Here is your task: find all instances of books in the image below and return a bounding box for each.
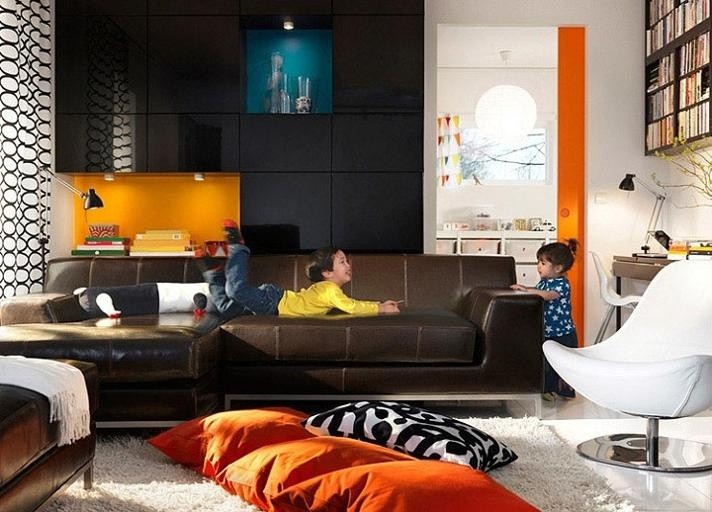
[646,0,710,151]
[667,239,712,261]
[71,230,195,256]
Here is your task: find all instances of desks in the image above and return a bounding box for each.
[611,254,677,333]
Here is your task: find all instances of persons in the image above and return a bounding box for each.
[191,218,400,320]
[510,238,580,402]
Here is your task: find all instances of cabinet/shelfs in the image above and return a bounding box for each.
[436,231,557,289]
[56,2,423,254]
[643,1,711,157]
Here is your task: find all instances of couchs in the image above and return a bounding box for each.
[0,254,545,428]
[0,354,97,511]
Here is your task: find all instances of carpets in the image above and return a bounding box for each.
[36,414,640,512]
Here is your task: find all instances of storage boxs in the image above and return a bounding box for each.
[504,239,544,262]
[461,239,500,254]
[89,223,120,238]
[437,238,457,254]
[515,265,542,287]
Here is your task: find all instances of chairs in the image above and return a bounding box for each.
[541,258,710,474]
[587,250,642,344]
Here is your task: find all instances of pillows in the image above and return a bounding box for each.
[214,435,420,512]
[297,397,519,472]
[146,406,317,479]
[267,458,540,511]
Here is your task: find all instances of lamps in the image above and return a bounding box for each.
[475,48,538,140]
[618,172,670,259]
[40,165,104,287]
